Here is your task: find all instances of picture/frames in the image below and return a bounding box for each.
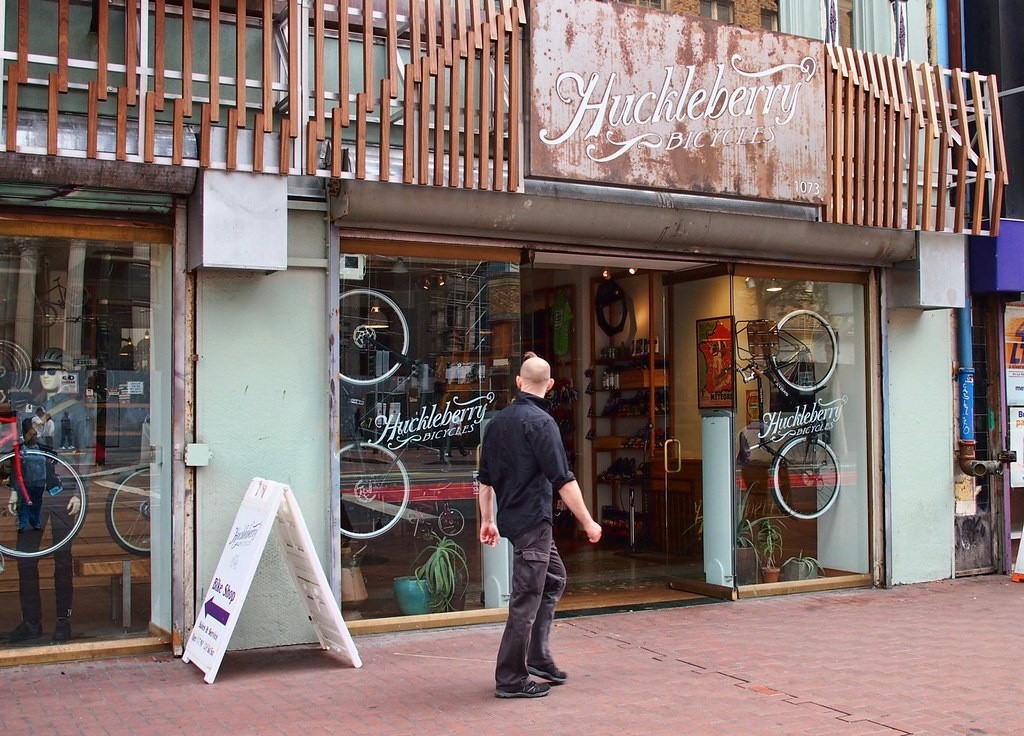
[695,315,737,408]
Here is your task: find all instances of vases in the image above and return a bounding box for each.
[342,566,369,620]
[446,574,470,610]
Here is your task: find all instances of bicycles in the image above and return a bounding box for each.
[406,481,465,540]
[23,276,90,328]
[0,393,88,561]
[105,465,151,557]
[715,309,841,522]
[340,288,465,541]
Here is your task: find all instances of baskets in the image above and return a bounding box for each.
[736,319,779,362]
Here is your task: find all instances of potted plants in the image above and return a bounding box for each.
[780,548,826,580]
[755,514,784,584]
[736,475,759,584]
[391,529,472,617]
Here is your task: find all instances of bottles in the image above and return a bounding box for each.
[603,372,619,390]
[601,336,659,358]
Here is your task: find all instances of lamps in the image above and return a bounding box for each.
[745,276,756,289]
[371,298,381,313]
[628,266,639,275]
[438,275,446,286]
[365,308,389,330]
[765,279,783,292]
[423,277,432,290]
[601,269,612,280]
[390,257,409,275]
[804,281,814,292]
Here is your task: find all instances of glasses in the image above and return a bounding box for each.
[37,368,68,376]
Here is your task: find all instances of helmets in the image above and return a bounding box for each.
[35,347,73,369]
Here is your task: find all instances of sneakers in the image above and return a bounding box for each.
[494,680,550,698]
[528,661,568,682]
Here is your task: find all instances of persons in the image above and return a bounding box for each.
[13,406,75,532]
[476,357,602,698]
[441,378,473,457]
[0,346,94,643]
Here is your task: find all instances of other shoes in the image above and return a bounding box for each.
[462,452,469,457]
[9,620,43,640]
[60,446,75,450]
[53,616,71,640]
[16,524,41,530]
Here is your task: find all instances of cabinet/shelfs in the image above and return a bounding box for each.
[647,453,817,564]
[590,353,670,548]
